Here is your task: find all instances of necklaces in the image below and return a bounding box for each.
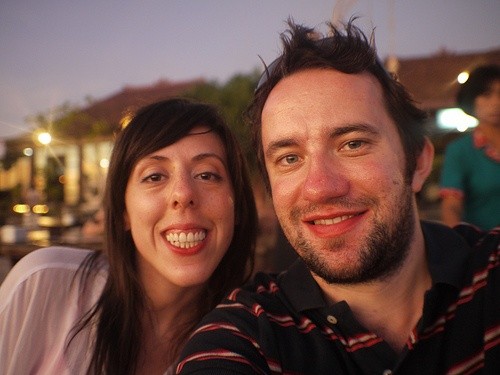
[139,289,203,326]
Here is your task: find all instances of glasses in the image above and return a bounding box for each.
[253,34,390,95]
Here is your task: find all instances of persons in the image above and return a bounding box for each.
[436,67,500,231]
[169,16,499,374]
[1,99,274,374]
